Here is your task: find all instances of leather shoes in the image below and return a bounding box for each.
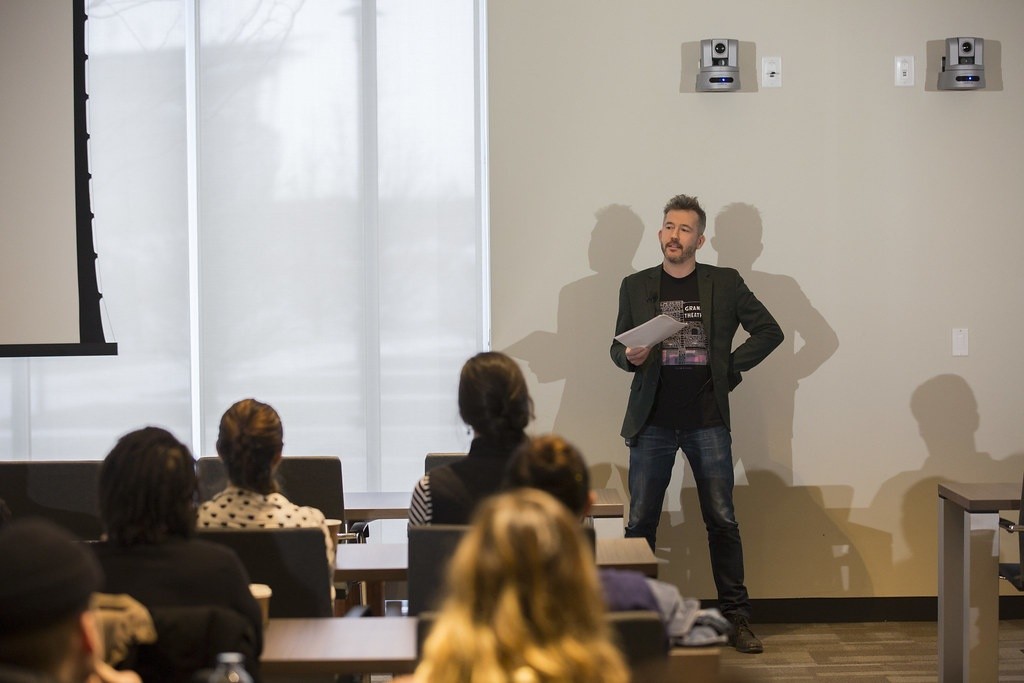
[724,609,767,655]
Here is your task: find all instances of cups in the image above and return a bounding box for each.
[248,584,272,631]
[327,519,342,555]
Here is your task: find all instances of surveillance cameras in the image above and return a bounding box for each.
[937,37,986,90]
[696,38,741,92]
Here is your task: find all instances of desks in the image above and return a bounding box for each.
[333,537,660,584]
[937,481,1024,683]
[343,487,626,525]
[258,617,722,683]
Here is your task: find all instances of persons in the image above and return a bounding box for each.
[406,352,530,534]
[85,427,264,683]
[500,437,660,612]
[196,398,336,610]
[0,521,141,683]
[609,193,784,655]
[413,488,628,682]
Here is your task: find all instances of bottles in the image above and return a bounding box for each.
[209,652,254,683]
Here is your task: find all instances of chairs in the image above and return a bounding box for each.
[0,459,105,540]
[118,605,262,683]
[195,526,334,618]
[196,457,346,533]
[415,608,670,683]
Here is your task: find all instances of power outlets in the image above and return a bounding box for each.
[893,55,914,87]
[762,55,782,88]
